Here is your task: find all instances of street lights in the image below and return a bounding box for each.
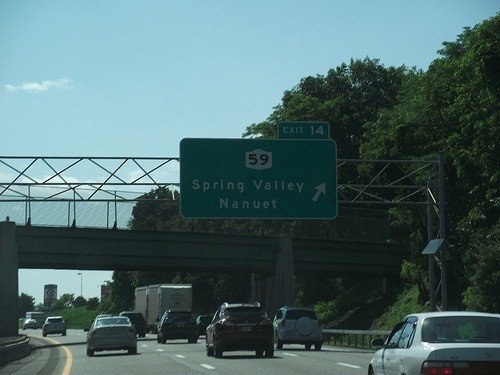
[77,273,83,297]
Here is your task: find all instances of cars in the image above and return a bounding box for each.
[196,316,212,338]
[119,312,146,337]
[155,309,199,344]
[84,316,137,356]
[206,302,274,358]
[368,312,500,375]
[43,316,67,337]
[23,318,37,330]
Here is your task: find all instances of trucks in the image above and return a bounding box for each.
[135,285,193,334]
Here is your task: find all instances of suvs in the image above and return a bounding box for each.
[273,305,323,351]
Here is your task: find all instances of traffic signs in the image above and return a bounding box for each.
[179,121,338,220]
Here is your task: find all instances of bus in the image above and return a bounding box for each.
[26,312,45,329]
